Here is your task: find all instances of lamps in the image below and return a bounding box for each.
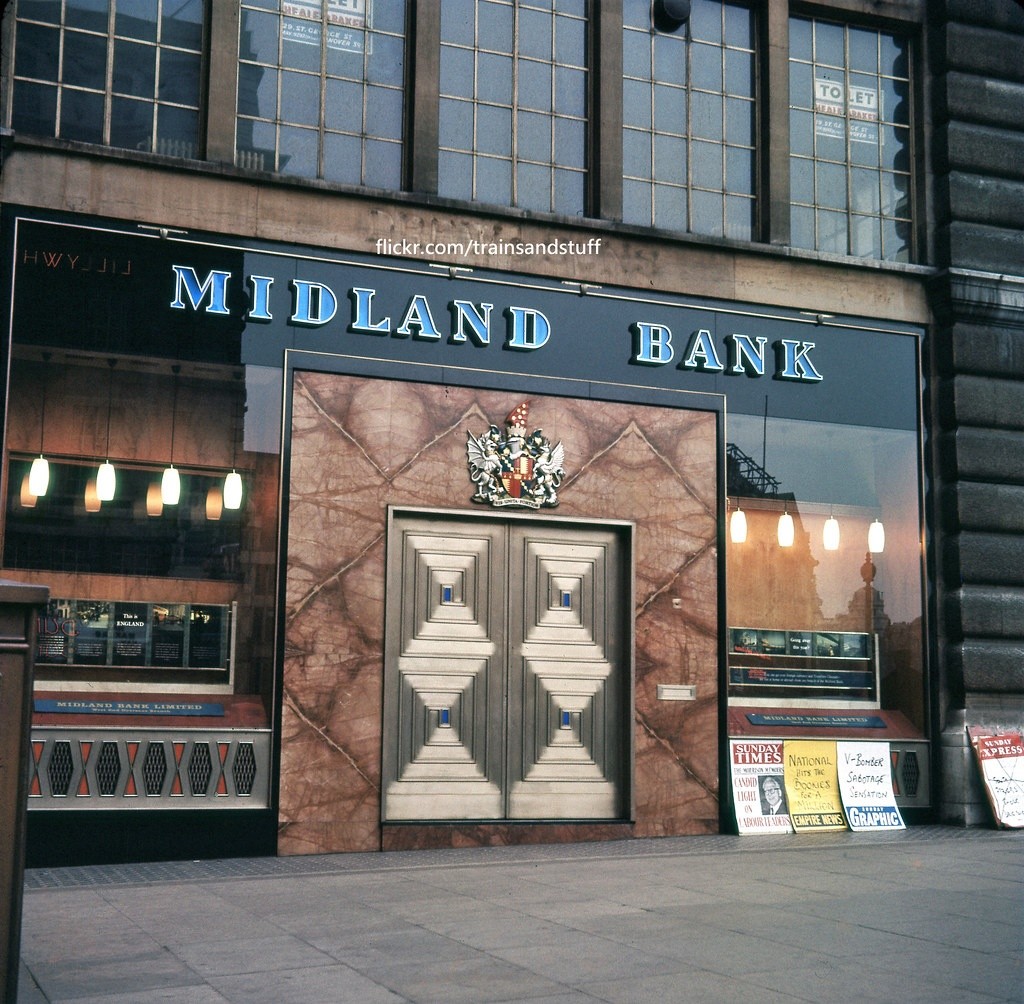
[730,491,887,554]
[16,352,247,521]
